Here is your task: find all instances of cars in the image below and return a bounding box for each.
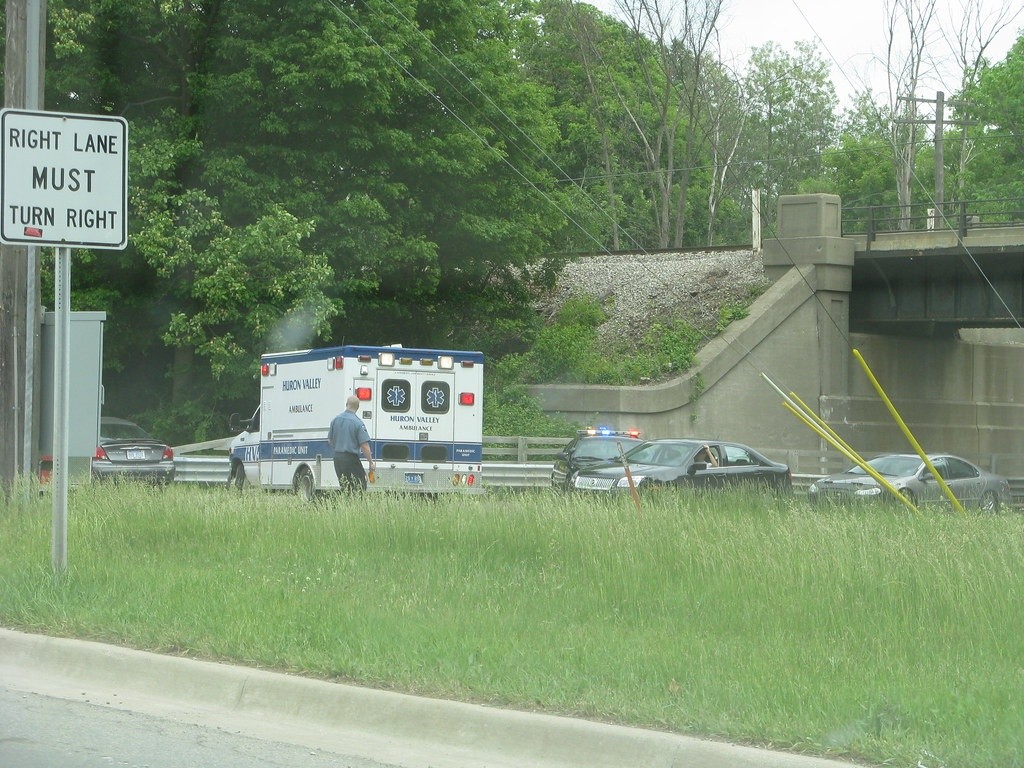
[93,416,175,486]
[570,438,793,506]
[551,426,645,494]
[808,455,1010,516]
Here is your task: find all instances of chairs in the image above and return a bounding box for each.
[737,458,751,466]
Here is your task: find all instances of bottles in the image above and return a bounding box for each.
[368,470,375,483]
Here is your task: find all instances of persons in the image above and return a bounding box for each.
[327,395,374,501]
[694,443,718,467]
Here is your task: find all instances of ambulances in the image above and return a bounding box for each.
[230,344,483,504]
